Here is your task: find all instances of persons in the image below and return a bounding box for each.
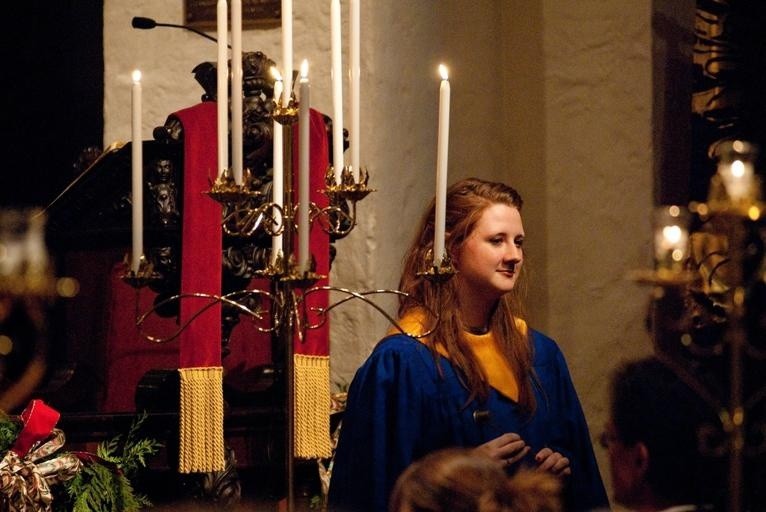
[325,178,609,512]
[390,447,563,512]
[154,51,347,181]
[600,358,731,512]
[153,154,174,181]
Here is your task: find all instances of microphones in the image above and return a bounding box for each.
[130,15,238,54]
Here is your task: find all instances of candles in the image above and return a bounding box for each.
[431,59,452,274]
[344,0,364,187]
[267,66,286,267]
[279,0,296,106]
[326,0,346,189]
[128,67,147,273]
[292,57,314,272]
[229,2,245,187]
[215,1,230,181]
